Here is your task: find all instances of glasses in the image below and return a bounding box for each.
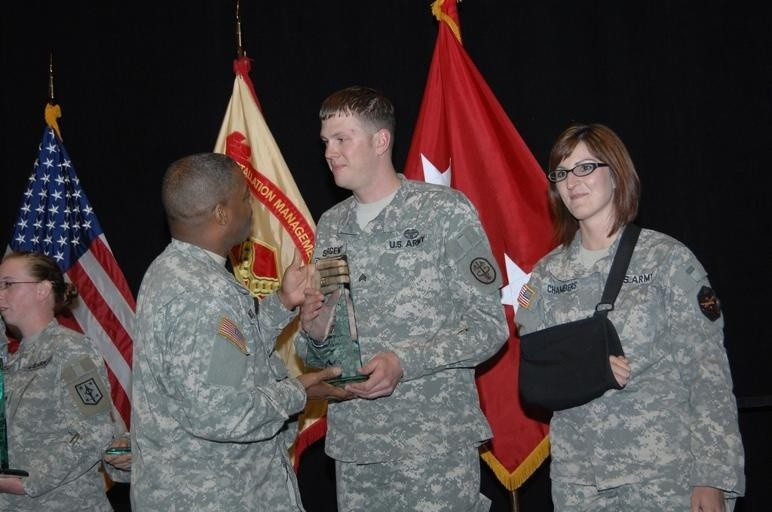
[547,163,609,183]
[0,281,42,289]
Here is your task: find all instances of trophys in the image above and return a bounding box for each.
[316,254,369,385]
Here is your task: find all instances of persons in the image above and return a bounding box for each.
[131,153,358,511]
[515,124,746,511]
[1,250,128,512]
[102,433,133,471]
[294,86,510,512]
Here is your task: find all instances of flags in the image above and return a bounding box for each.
[213,56,327,475]
[404,0,561,491]
[3,104,137,493]
